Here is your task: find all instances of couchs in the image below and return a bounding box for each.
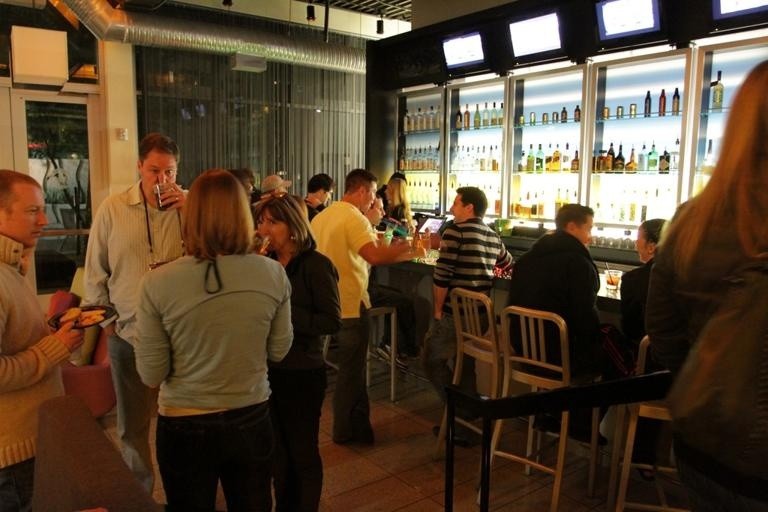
[59,331,116,417]
[31,393,163,512]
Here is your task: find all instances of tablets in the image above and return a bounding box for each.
[416,216,447,235]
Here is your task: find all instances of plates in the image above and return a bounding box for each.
[48,305,117,333]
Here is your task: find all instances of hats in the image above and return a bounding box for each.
[259,174,292,197]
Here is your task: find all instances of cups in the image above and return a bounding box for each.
[152,183,176,211]
[604,270,623,292]
[245,231,275,256]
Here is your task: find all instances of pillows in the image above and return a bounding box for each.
[68,324,100,365]
[46,292,81,314]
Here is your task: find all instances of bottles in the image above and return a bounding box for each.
[457,105,463,130]
[543,113,547,123]
[625,148,636,174]
[491,103,498,127]
[413,227,422,250]
[422,227,431,250]
[644,91,651,117]
[463,104,470,130]
[712,70,723,113]
[535,143,543,171]
[671,87,680,116]
[400,145,442,171]
[561,143,570,169]
[572,151,579,170]
[474,104,480,129]
[637,144,648,173]
[658,150,669,174]
[422,107,428,130]
[552,111,557,122]
[529,114,535,126]
[561,107,568,122]
[659,89,665,116]
[402,182,440,205]
[434,105,440,128]
[614,144,624,173]
[407,107,416,135]
[617,106,622,119]
[426,105,436,128]
[518,116,524,123]
[669,137,681,168]
[605,142,615,173]
[552,144,560,170]
[545,145,553,171]
[700,139,714,174]
[414,107,423,130]
[513,187,578,219]
[450,145,498,171]
[597,150,606,173]
[482,103,489,128]
[648,144,658,174]
[574,105,581,121]
[446,182,501,215]
[403,109,409,134]
[602,107,610,119]
[499,103,503,126]
[526,144,534,169]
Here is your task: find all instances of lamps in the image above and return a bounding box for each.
[306,5,314,22]
[378,21,382,34]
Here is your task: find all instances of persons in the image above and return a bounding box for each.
[138,170,291,512]
[2,169,86,512]
[302,173,336,221]
[602,218,691,483]
[375,172,408,200]
[644,60,768,512]
[257,194,341,512]
[509,204,623,445]
[230,168,262,202]
[364,194,386,225]
[385,179,416,232]
[423,188,514,450]
[84,134,188,497]
[310,170,426,452]
[259,176,298,202]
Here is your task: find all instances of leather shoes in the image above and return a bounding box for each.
[433,426,470,449]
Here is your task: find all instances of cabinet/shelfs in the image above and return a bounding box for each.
[687,36,768,199]
[585,49,693,253]
[508,64,587,223]
[394,86,445,215]
[443,76,509,219]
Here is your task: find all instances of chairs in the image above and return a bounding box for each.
[364,305,398,403]
[476,306,600,512]
[607,336,692,512]
[432,287,513,474]
